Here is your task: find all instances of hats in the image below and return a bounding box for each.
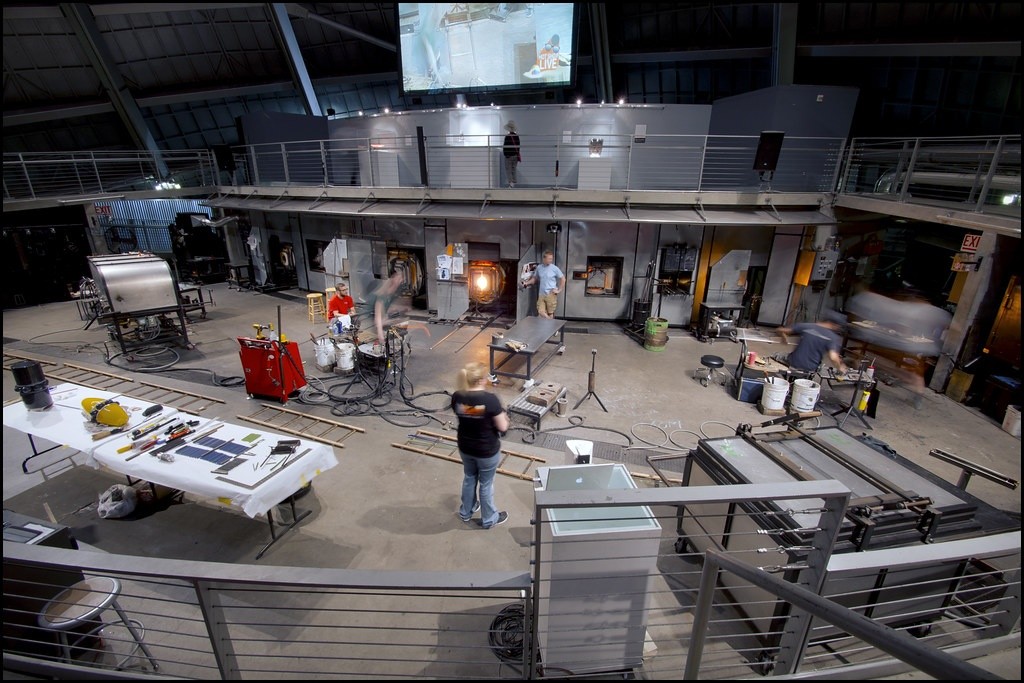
[824,309,859,330]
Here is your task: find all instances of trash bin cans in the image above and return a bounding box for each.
[644,317,668,352]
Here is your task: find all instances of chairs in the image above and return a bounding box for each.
[733,339,779,392]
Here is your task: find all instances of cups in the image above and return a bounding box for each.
[373,345,378,355]
[866,366,875,379]
[749,352,757,365]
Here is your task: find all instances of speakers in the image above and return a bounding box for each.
[213,144,237,171]
[753,131,785,170]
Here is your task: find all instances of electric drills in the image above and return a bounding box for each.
[252,324,269,340]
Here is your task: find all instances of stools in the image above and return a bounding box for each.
[693,355,727,387]
[307,293,326,324]
[36,576,159,672]
[325,287,336,322]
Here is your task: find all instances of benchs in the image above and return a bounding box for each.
[506,379,567,431]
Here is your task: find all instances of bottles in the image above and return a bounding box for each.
[328,319,343,337]
[858,386,873,410]
[388,358,391,368]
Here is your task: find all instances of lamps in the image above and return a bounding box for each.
[57,194,125,203]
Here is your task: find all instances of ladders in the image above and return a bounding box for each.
[236,403,365,448]
[121,381,224,415]
[3,352,57,371]
[43,363,134,391]
[391,429,546,481]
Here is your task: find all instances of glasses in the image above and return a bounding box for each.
[339,288,348,291]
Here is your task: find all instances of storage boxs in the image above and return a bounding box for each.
[135,481,172,503]
[944,368,976,404]
[718,319,734,329]
[736,376,764,404]
[1001,404,1022,440]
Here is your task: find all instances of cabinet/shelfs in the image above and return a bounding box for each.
[178,283,207,325]
[223,261,253,292]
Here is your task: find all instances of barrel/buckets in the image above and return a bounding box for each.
[761,377,790,410]
[492,332,504,344]
[945,369,975,404]
[790,378,821,413]
[643,316,668,352]
[334,343,355,370]
[632,299,652,326]
[313,339,335,367]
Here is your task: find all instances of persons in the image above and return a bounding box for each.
[503,118,521,188]
[451,360,510,527]
[771,316,848,376]
[327,282,361,334]
[168,222,187,266]
[520,248,567,337]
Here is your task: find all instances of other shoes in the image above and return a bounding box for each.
[554,331,558,336]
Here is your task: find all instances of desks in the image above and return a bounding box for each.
[817,365,877,418]
[93,411,340,561]
[840,321,940,377]
[983,374,1022,422]
[3,381,179,516]
[697,301,746,343]
[487,315,568,389]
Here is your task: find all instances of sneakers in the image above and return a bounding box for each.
[482,511,509,528]
[461,501,481,521]
